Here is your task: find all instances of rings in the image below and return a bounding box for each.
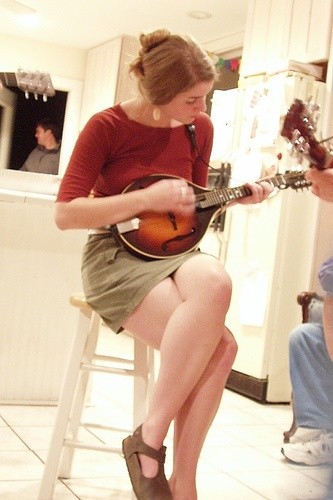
[180,188,187,197]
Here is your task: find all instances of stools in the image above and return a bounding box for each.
[37,293,155,500]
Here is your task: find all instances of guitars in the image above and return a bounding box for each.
[277,94,333,171]
[108,169,313,264]
[1,65,57,103]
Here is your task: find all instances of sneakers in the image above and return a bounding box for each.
[281,433,333,466]
[288,427,325,442]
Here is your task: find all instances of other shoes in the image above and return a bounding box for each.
[122,423,173,500]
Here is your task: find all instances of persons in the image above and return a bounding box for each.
[19,117,63,176]
[281,257,333,465]
[54,25,276,500]
[306,167,333,203]
